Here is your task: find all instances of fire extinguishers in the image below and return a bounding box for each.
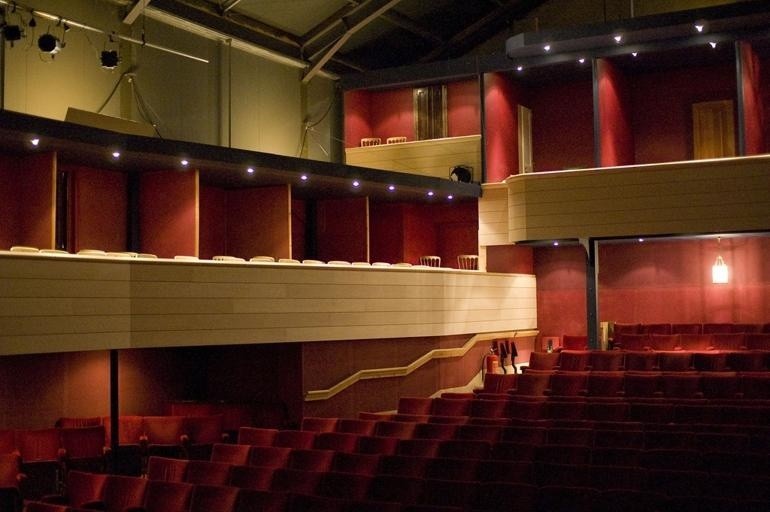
[482,347,499,382]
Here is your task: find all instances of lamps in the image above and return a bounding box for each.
[1,0,210,71]
[712,237,728,284]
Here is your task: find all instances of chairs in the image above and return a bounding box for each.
[1,244,479,274]
[361,137,381,147]
[0,322,770,512]
[386,136,407,144]
